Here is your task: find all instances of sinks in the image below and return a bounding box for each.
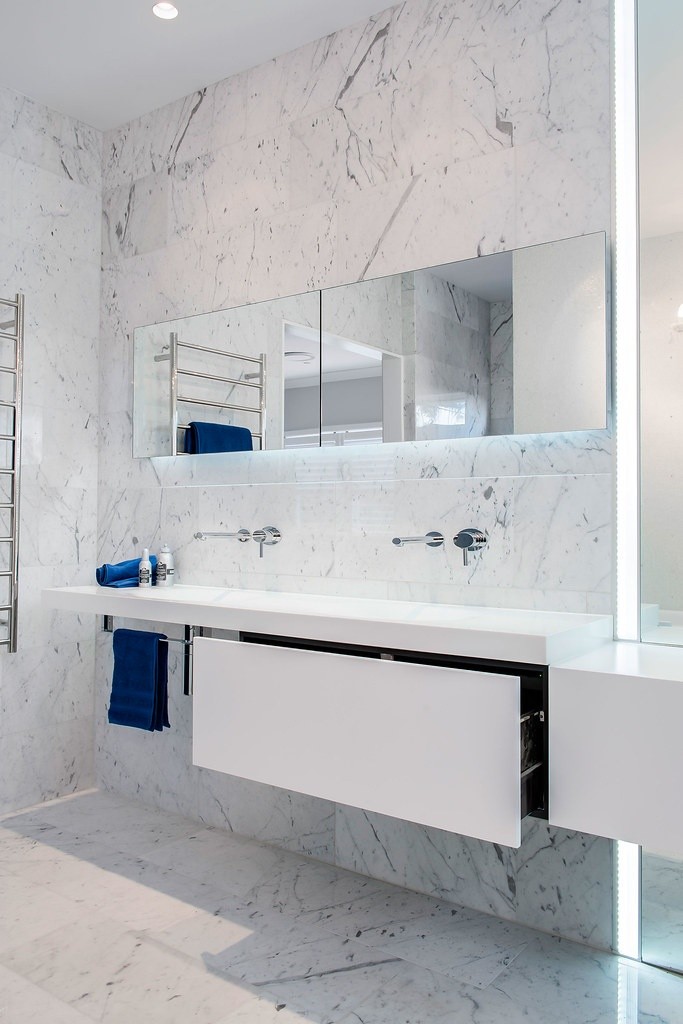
[131,582,613,666]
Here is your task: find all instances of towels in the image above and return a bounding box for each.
[95,554,158,588]
[184,421,253,454]
[108,628,171,733]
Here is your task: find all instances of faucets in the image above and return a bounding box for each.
[194,529,251,542]
[391,531,445,547]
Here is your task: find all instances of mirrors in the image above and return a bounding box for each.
[130,229,607,460]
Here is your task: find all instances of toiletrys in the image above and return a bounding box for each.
[156,543,175,587]
[139,548,152,590]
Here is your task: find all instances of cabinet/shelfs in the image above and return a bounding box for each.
[190,627,551,848]
